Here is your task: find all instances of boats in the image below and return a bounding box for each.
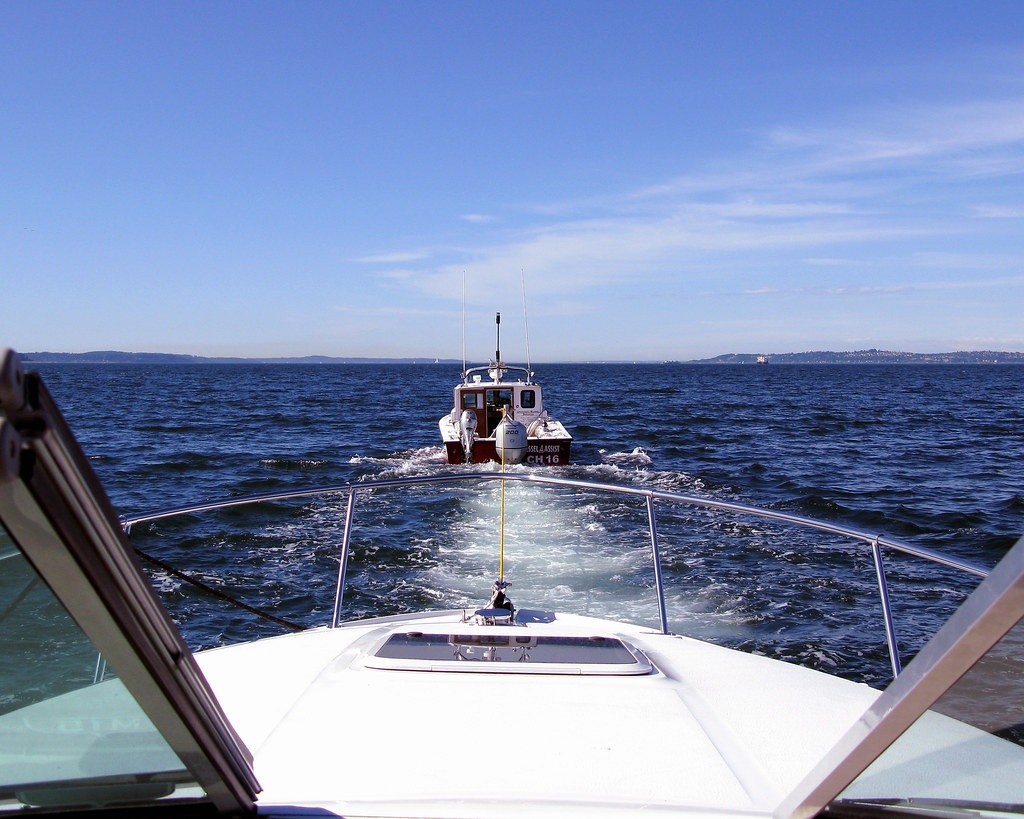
[441,271,574,467]
[756,356,770,364]
